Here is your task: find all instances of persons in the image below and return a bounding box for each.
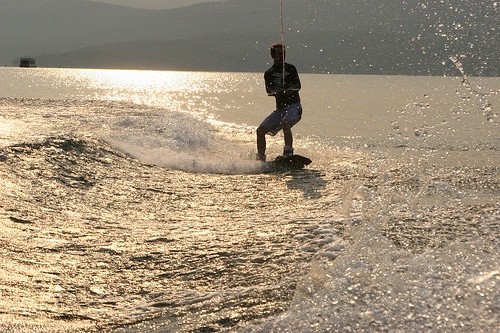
[255,44,302,161]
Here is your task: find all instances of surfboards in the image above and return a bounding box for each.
[261,154,312,170]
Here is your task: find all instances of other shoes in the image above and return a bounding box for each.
[255,153,266,162]
[275,147,294,160]
